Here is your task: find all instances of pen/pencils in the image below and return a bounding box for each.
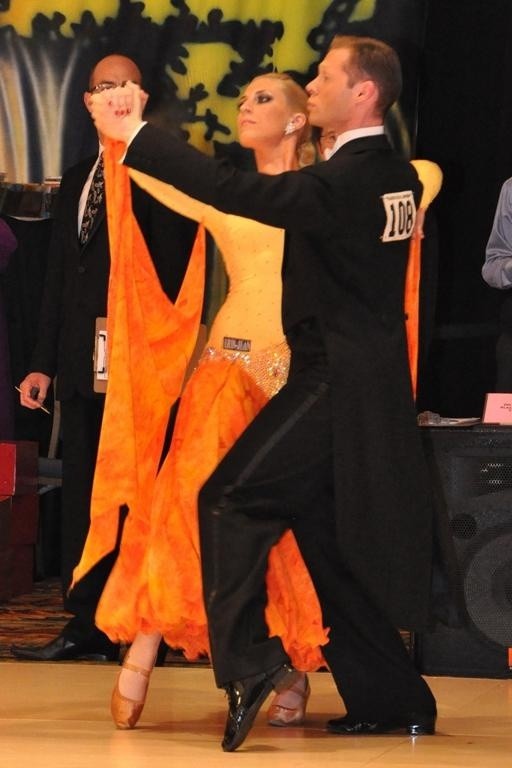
[15,386,51,415]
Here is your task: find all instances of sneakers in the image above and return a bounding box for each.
[110,646,158,730]
[266,673,310,727]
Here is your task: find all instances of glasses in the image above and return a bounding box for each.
[89,80,137,93]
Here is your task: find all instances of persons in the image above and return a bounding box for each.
[481,177,512,394]
[10,52,215,669]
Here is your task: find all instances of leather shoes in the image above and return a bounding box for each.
[10,634,117,662]
[221,660,298,752]
[325,704,436,735]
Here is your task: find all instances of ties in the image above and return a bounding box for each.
[79,150,105,247]
